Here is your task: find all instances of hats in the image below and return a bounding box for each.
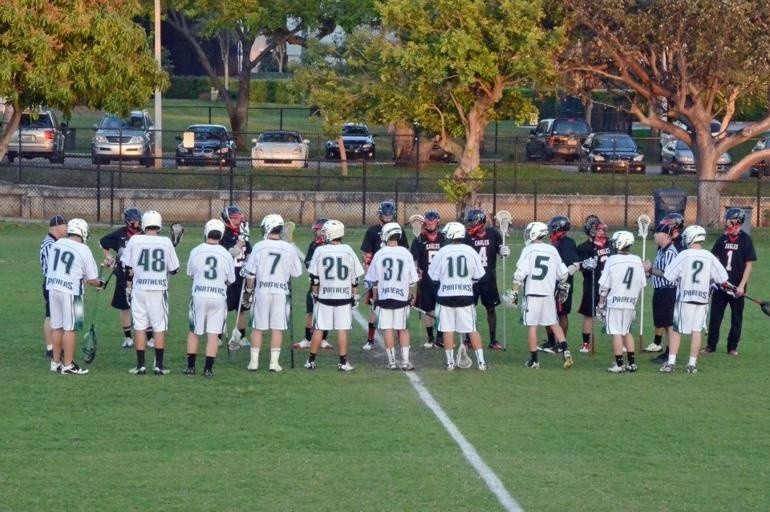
[49,215,68,226]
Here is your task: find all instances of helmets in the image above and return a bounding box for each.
[659,213,685,234]
[723,207,746,226]
[611,231,636,250]
[122,207,142,227]
[522,221,549,246]
[260,214,284,239]
[312,218,345,244]
[220,206,244,226]
[680,225,706,246]
[67,217,91,243]
[648,224,673,236]
[379,222,402,243]
[548,216,607,245]
[421,208,487,241]
[204,218,225,240]
[377,200,397,219]
[140,210,163,232]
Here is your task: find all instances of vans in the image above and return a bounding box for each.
[525,117,593,165]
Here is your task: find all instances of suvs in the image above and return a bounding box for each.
[91,110,155,167]
[5,106,72,164]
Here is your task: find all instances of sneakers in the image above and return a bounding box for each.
[121,337,740,377]
[45,349,65,358]
[60,360,89,376]
[49,357,63,372]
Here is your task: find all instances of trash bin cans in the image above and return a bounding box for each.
[62,128,76,149]
[652,188,687,232]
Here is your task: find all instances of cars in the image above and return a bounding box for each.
[661,137,732,175]
[659,118,721,147]
[251,131,310,170]
[578,131,646,175]
[391,119,452,161]
[325,123,378,161]
[174,124,238,169]
[749,137,770,176]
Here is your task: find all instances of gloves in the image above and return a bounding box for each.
[310,292,319,304]
[240,287,254,310]
[595,302,607,324]
[507,289,519,309]
[125,288,133,306]
[641,257,652,279]
[96,280,106,291]
[584,255,598,271]
[567,262,581,277]
[499,244,510,257]
[554,283,572,304]
[103,254,119,269]
[351,293,362,310]
